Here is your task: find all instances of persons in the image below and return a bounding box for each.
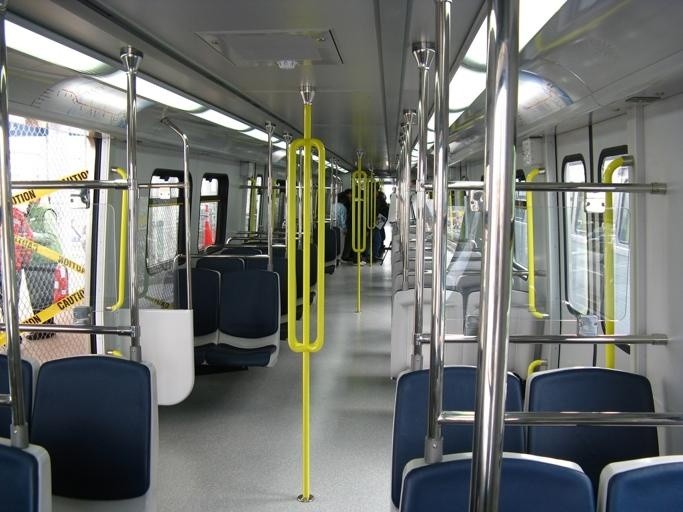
[329,179,419,267]
[0,205,33,347]
[16,196,64,340]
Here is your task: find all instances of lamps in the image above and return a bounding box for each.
[392,0,567,168]
